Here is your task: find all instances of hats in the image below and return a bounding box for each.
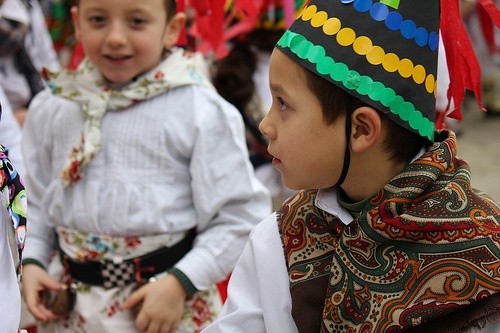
[276,0,441,142]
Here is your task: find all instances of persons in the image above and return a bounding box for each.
[22,0,272,333]
[197,0,500,333]
[0,0,500,197]
